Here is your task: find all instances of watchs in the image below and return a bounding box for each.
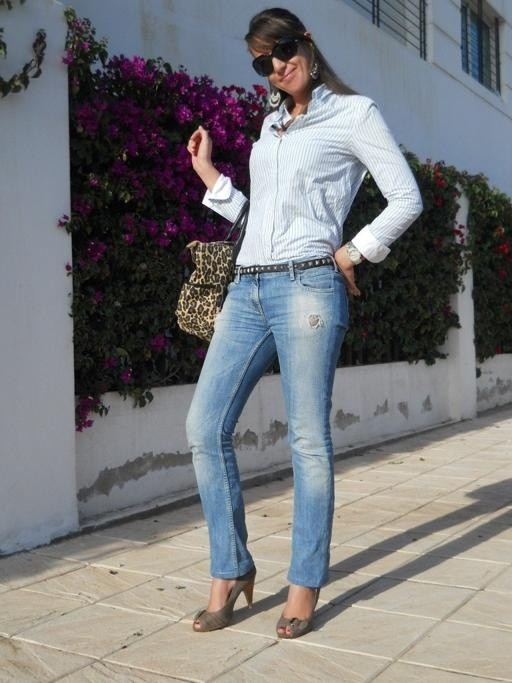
[344,241,363,265]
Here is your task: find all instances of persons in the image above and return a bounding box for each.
[184,7,423,639]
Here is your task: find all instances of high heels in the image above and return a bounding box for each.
[193,565,257,631]
[276,586,321,639]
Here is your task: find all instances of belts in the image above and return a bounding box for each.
[234,257,334,274]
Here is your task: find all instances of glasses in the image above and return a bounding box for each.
[252,38,301,77]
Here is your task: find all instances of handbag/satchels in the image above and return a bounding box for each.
[175,200,250,342]
